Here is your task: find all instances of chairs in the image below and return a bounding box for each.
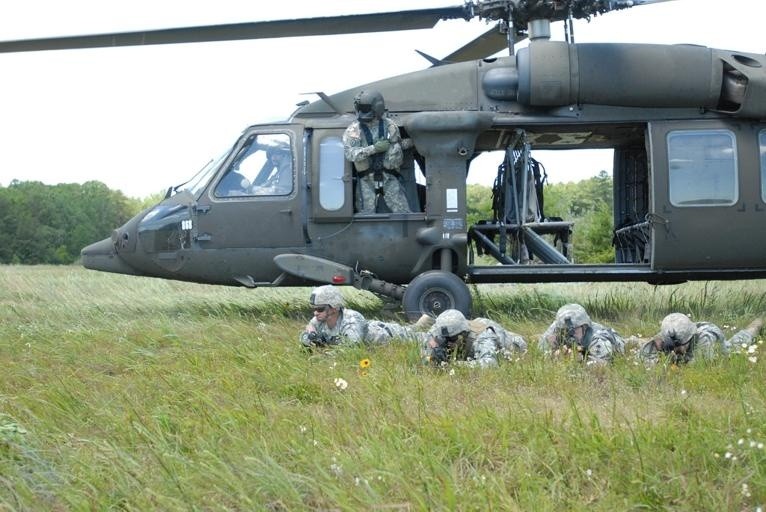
[468,145,575,264]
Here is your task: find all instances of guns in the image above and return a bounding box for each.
[306,333,340,348]
[663,328,690,365]
[560,315,575,350]
[431,342,449,368]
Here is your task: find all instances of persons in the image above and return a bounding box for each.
[537,303,642,368]
[420,309,528,375]
[300,284,436,356]
[342,88,414,214]
[241,140,293,197]
[640,312,766,364]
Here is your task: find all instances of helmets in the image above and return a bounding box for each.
[355,88,385,120]
[661,312,698,347]
[310,285,346,311]
[435,309,470,337]
[555,302,593,330]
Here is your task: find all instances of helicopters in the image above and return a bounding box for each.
[0,0,766,325]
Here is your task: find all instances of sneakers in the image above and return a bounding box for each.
[415,313,434,328]
[745,317,764,336]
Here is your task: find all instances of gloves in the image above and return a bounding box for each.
[374,135,390,153]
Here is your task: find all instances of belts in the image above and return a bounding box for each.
[357,167,399,177]
[371,321,395,339]
